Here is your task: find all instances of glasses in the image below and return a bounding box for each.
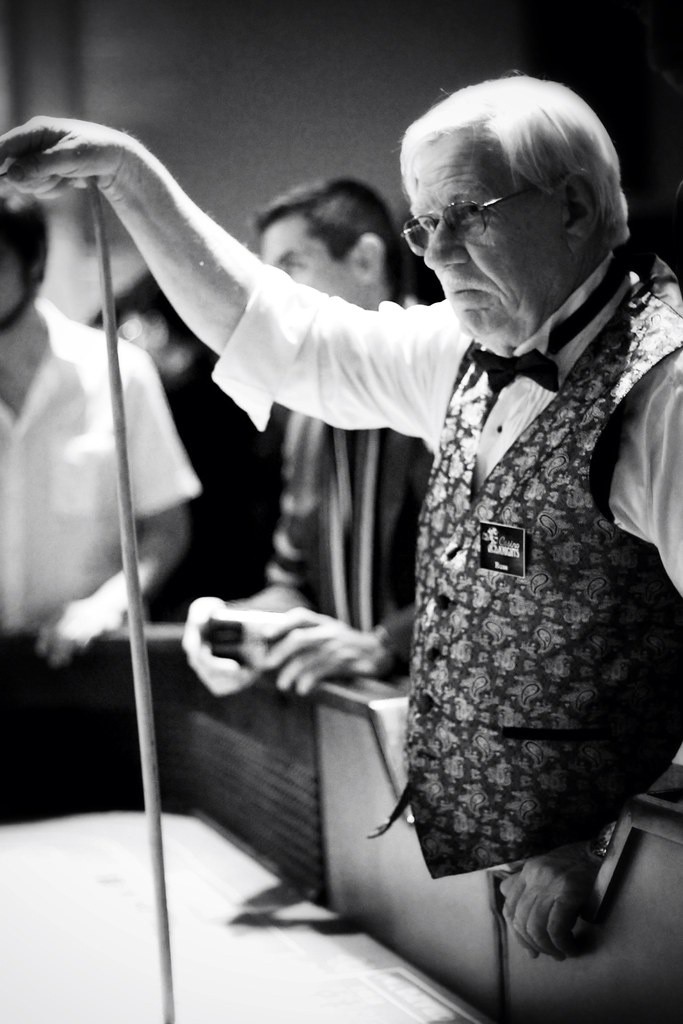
[400,171,568,257]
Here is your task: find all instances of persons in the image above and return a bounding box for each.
[0,76,683,961]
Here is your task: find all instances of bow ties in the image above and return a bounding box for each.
[471,348,559,393]
